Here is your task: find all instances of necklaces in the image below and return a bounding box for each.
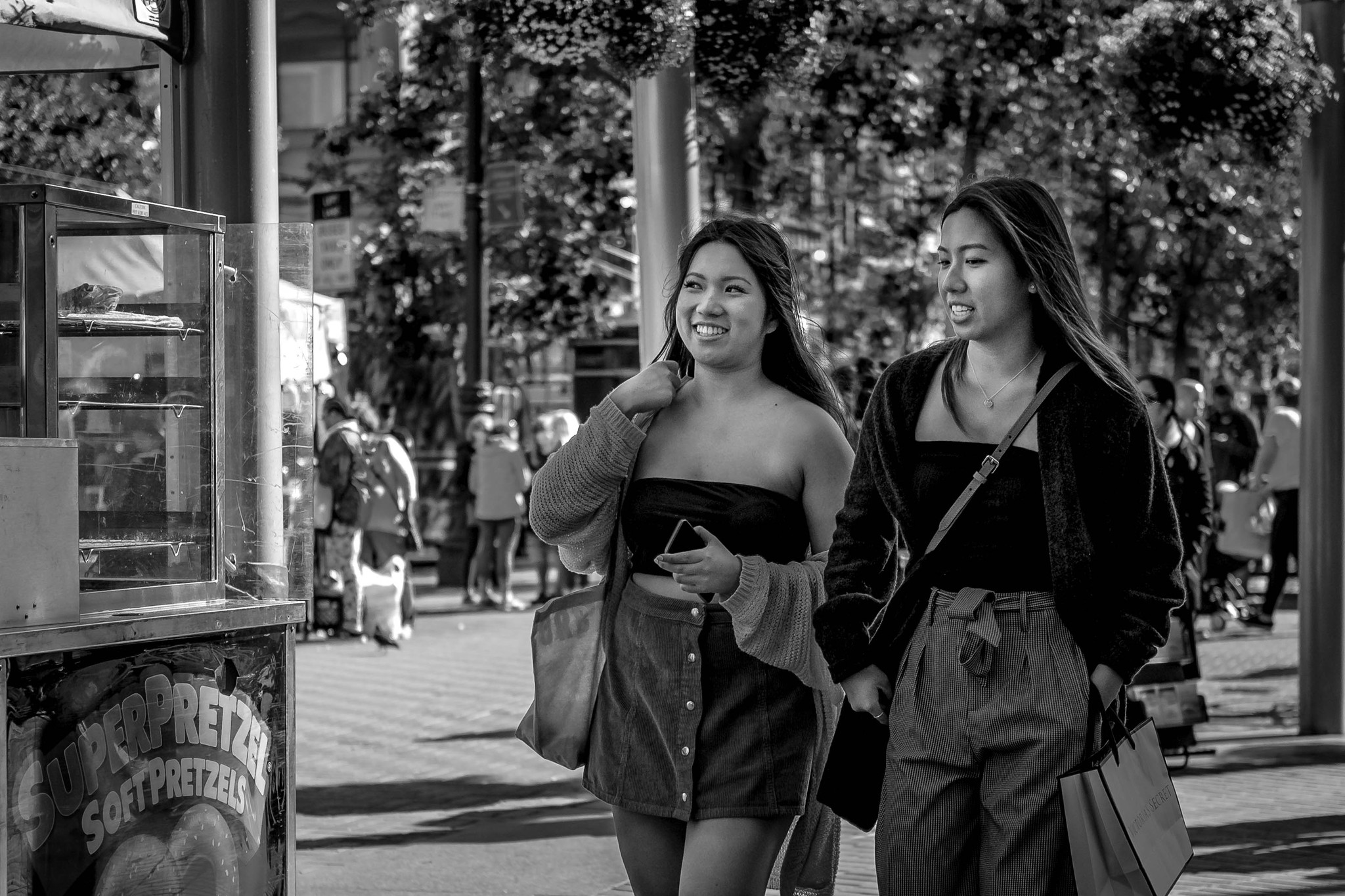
[966,347,1041,409]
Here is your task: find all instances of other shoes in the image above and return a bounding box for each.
[1237,614,1273,631]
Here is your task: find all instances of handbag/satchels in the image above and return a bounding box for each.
[362,549,405,639]
[817,665,890,831]
[312,467,333,528]
[400,509,422,551]
[314,586,344,636]
[1056,678,1193,896]
[336,483,359,524]
[513,575,609,769]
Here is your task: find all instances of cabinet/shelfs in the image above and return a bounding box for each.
[0,179,227,612]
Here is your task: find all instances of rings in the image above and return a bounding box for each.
[873,712,884,718]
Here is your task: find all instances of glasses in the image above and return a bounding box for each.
[1144,394,1157,403]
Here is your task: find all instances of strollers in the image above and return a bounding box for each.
[1190,474,1279,633]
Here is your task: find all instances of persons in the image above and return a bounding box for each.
[451,407,590,610]
[310,391,418,645]
[813,173,1186,896]
[1134,375,1301,632]
[829,354,889,421]
[528,217,856,896]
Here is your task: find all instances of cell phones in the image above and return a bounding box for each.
[664,518,716,604]
